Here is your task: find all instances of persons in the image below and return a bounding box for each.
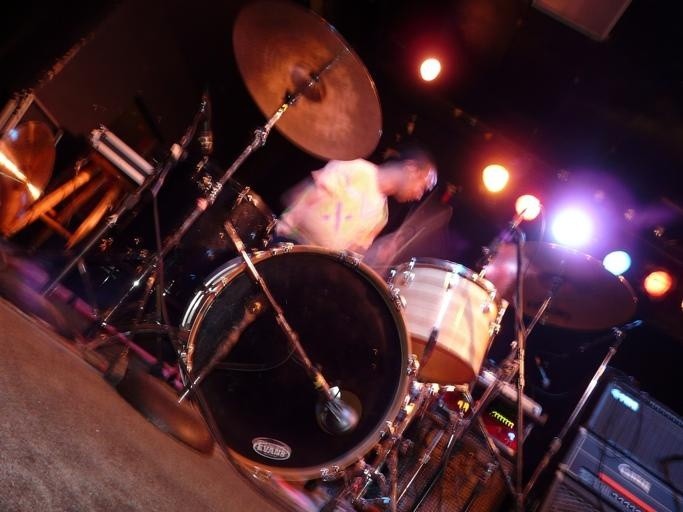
[150,137,441,396]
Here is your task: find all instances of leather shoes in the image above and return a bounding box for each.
[149,363,170,382]
[168,376,183,393]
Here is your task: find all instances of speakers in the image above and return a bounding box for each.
[493,419,683,512]
[586,379,682,495]
[388,406,505,510]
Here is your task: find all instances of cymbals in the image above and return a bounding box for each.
[484,241,637,333]
[0,120,55,232]
[232,0,383,159]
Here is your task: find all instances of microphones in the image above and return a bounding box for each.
[491,210,525,252]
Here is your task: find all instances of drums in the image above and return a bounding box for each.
[124,156,280,283]
[175,240,419,481]
[387,256,502,386]
